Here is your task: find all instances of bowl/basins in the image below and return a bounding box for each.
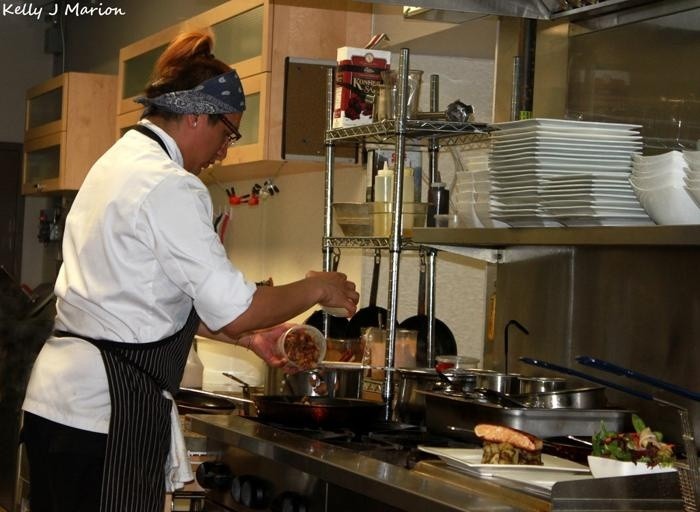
[174,390,235,415]
[505,385,608,408]
[517,376,566,394]
[462,368,520,394]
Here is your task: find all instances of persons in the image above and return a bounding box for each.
[18,31,360,512]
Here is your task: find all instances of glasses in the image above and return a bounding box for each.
[219,114,242,148]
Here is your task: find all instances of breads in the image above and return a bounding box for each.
[474,422,544,453]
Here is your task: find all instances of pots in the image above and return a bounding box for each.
[250,392,394,428]
[303,252,351,340]
[348,254,400,339]
[399,255,457,368]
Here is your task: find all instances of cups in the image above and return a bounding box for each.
[382,70,424,121]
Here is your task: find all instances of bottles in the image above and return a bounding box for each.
[181,344,204,388]
[276,324,327,371]
[427,171,451,227]
[404,157,416,237]
[372,161,394,238]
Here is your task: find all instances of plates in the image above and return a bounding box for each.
[492,471,594,496]
[587,453,678,479]
[454,118,700,228]
[417,444,590,477]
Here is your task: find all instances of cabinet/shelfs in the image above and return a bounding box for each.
[113,0,378,184]
[21,72,117,199]
[392,0,699,251]
